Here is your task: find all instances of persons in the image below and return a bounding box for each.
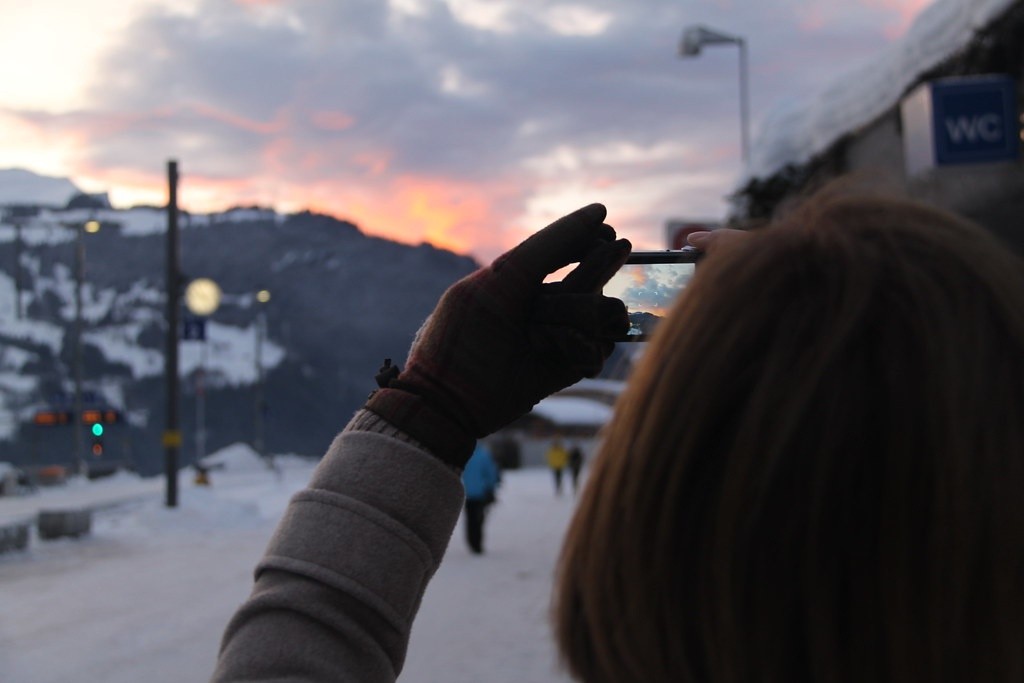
[210,167,1024,683]
[547,434,566,490]
[567,439,584,489]
[461,438,498,554]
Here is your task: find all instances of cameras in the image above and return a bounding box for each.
[600,246,703,342]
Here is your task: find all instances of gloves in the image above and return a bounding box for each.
[364,202,632,470]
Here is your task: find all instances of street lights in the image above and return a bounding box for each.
[678,25,752,172]
[73,215,100,477]
[255,286,271,457]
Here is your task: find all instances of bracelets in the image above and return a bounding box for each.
[374,357,471,425]
